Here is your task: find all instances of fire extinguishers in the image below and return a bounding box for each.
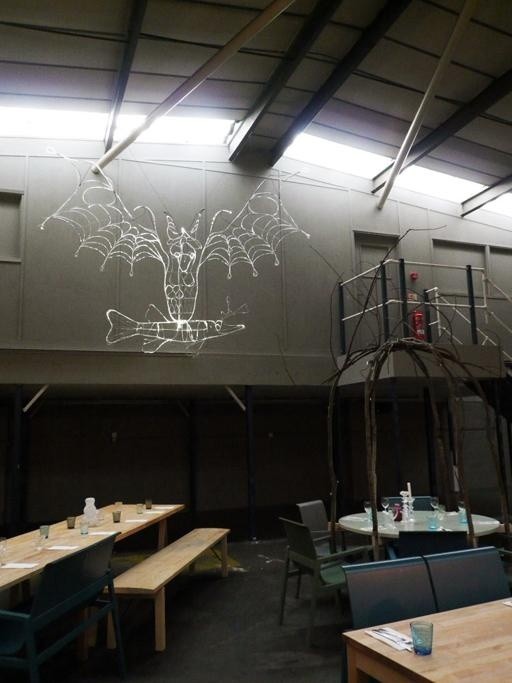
[406,311,426,341]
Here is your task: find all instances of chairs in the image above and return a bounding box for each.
[423,546,511,612]
[0,573,128,682]
[396,531,468,558]
[295,499,352,599]
[384,492,433,511]
[341,556,438,629]
[277,512,357,646]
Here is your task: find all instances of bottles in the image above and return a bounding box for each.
[83,498,98,526]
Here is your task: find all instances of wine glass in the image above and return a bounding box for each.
[364,497,465,531]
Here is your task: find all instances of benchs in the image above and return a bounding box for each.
[105,522,230,650]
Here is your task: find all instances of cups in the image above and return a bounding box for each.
[0,537,10,566]
[39,516,89,541]
[411,621,434,657]
[98,500,153,523]
[31,535,46,552]
